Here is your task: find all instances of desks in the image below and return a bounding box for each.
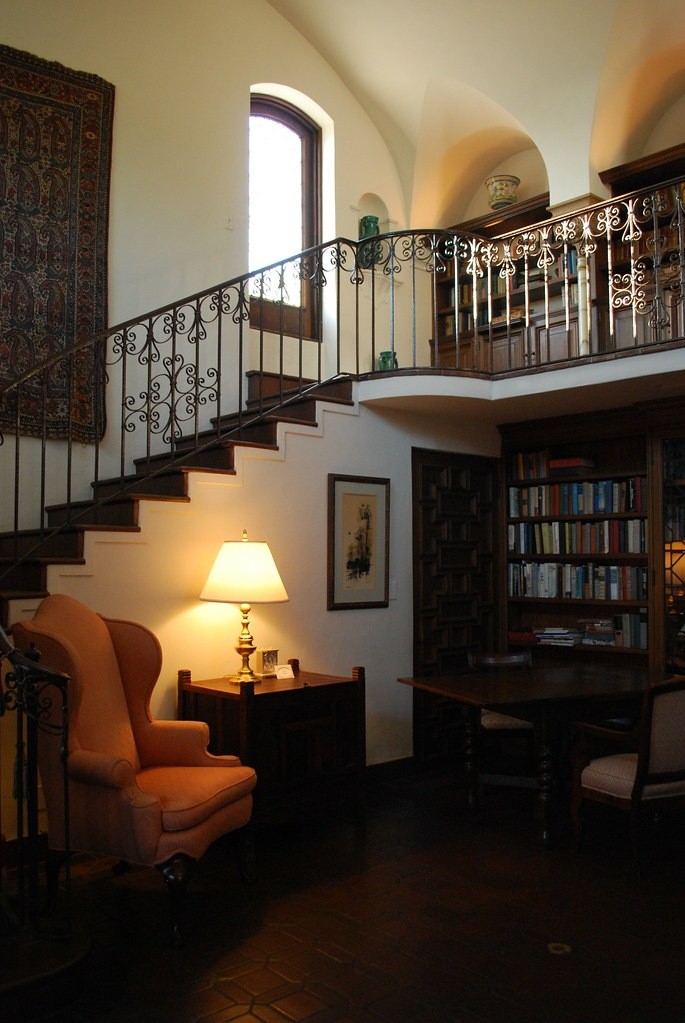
[396,667,671,859]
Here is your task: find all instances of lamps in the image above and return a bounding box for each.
[198,529,290,685]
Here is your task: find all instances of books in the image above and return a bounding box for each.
[508,519,648,554]
[512,447,595,481]
[507,560,648,600]
[509,476,647,518]
[615,182,685,293]
[508,607,648,649]
[445,224,578,337]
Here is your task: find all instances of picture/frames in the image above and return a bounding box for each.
[326,472,389,612]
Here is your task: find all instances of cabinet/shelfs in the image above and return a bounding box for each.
[423,191,579,372]
[494,395,685,674]
[597,143,685,351]
[176,658,368,891]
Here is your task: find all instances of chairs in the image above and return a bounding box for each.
[10,593,258,951]
[569,677,685,880]
[470,644,537,788]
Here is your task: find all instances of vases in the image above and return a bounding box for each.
[484,175,521,210]
